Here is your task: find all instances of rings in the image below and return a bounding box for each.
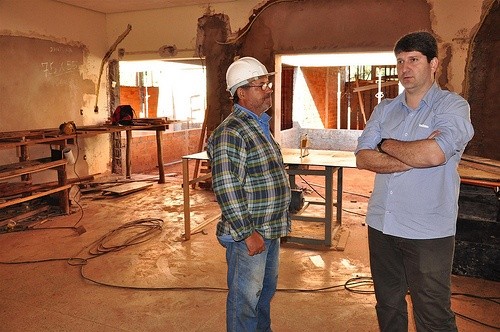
[255,252,258,255]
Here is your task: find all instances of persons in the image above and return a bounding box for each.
[207,57,293,332]
[355,32,474,332]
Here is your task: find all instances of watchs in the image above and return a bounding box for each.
[377,138,386,154]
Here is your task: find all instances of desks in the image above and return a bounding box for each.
[181,150,343,247]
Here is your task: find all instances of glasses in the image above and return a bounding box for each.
[240,82,273,91]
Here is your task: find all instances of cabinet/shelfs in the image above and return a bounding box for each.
[0,134,71,227]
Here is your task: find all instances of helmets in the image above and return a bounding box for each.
[225,57,278,91]
